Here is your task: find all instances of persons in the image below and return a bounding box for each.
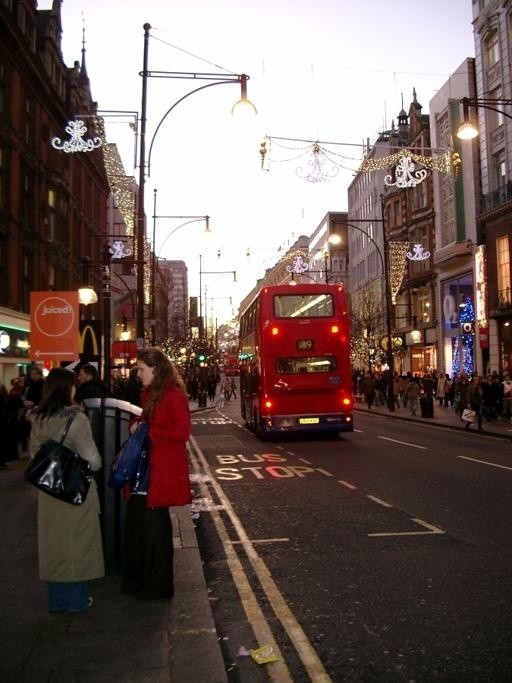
[123,349,191,599]
[177,357,192,393]
[109,366,142,407]
[1,368,45,464]
[353,367,512,431]
[193,366,237,402]
[72,362,108,402]
[25,367,103,613]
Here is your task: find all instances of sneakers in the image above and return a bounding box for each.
[88,597,94,608]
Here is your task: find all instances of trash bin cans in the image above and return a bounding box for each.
[199,393,206,407]
[421,398,433,418]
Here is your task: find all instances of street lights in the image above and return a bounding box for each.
[137,22,258,356]
[327,214,395,412]
[456,97,510,140]
[151,187,213,347]
[199,248,222,339]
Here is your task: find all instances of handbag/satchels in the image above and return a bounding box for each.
[128,440,152,496]
[461,409,477,423]
[25,441,95,507]
[108,421,149,490]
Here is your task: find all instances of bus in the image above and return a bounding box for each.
[239,282,356,443]
